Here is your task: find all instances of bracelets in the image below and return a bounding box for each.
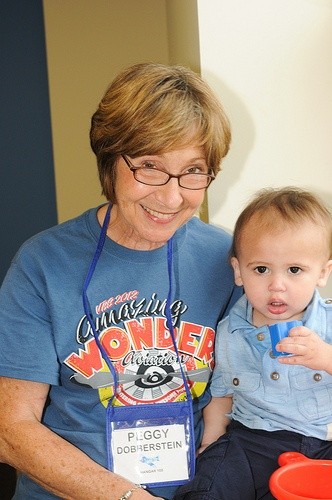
[117,484,146,500]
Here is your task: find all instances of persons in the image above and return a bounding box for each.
[0,59,245,500]
[173,186,332,500]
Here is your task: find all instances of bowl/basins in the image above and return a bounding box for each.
[268,451,332,500]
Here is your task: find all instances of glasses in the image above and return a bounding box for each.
[121,153,215,190]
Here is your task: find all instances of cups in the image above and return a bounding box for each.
[269,321,304,355]
